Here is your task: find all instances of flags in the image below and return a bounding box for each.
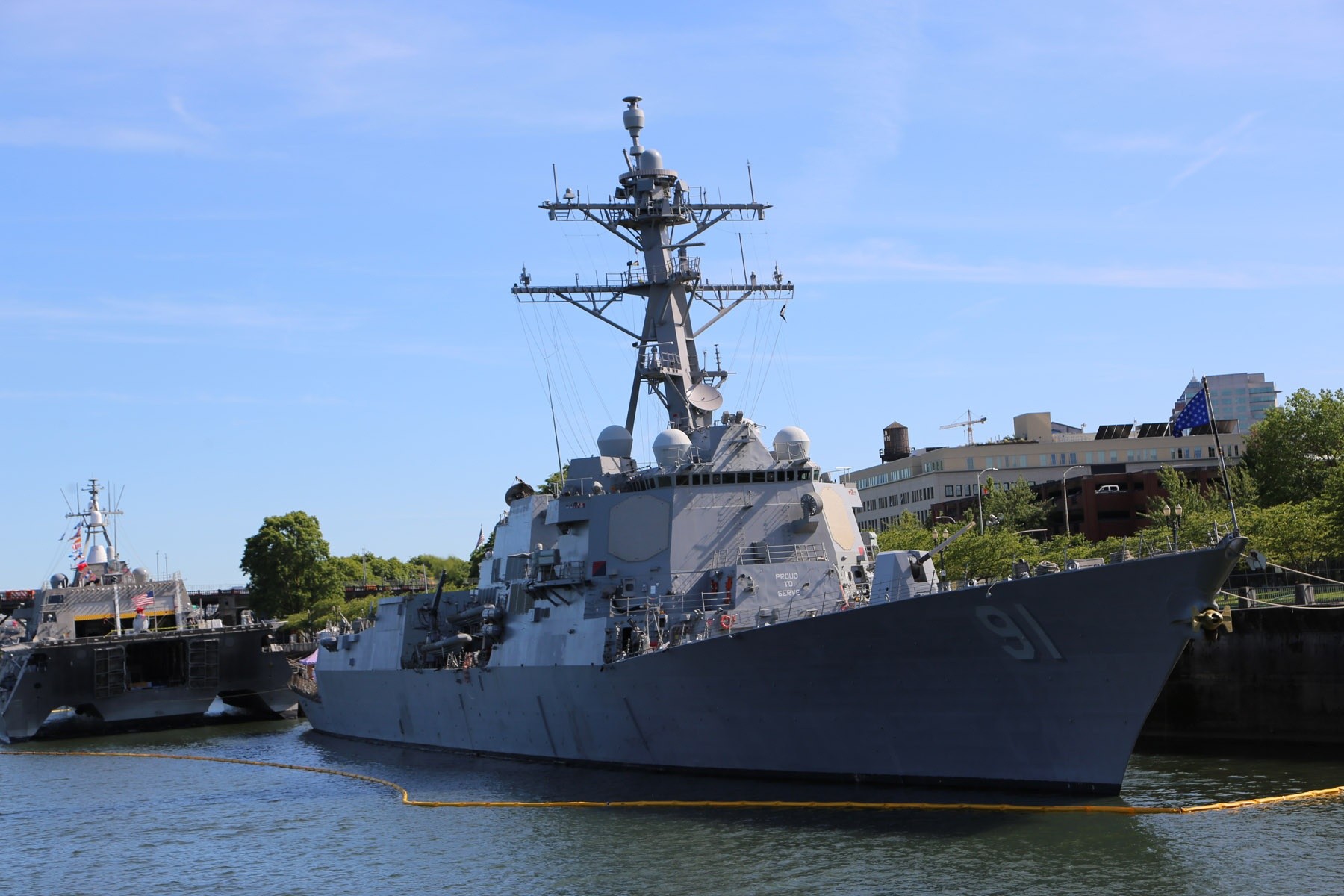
[131,590,155,606]
[1172,388,1211,438]
[67,526,96,581]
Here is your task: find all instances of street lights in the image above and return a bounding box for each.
[1163,504,1184,555]
[934,531,950,581]
[331,604,342,627]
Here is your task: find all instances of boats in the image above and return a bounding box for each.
[293,95,1253,825]
[1,477,314,746]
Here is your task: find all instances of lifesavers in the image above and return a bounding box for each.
[720,614,734,629]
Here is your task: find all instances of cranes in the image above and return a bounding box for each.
[940,408,988,446]
